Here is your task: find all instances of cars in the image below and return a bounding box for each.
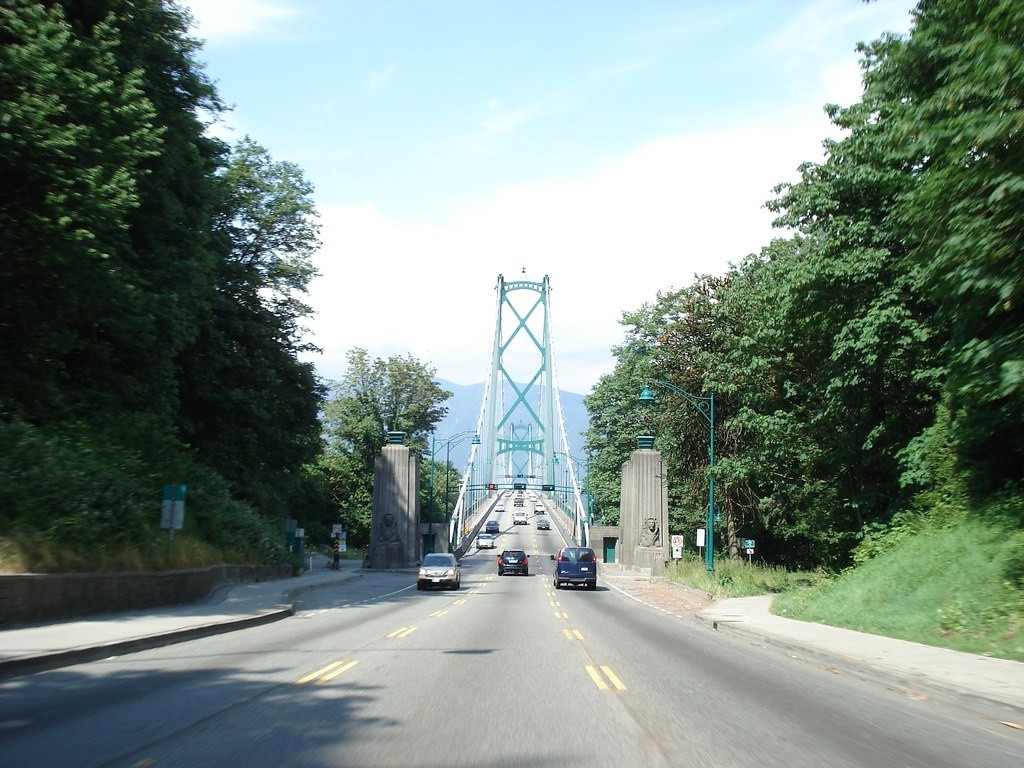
[495,503,505,512]
[475,533,496,549]
[416,553,462,591]
[536,518,551,529]
[533,504,545,514]
[529,494,538,502]
[504,488,531,499]
[485,520,501,533]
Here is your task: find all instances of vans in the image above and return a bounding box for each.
[549,546,596,591]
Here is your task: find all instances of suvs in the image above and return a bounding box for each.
[513,497,524,507]
[511,511,529,525]
[495,548,530,576]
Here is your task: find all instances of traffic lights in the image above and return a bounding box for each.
[486,483,498,490]
[542,485,555,491]
[514,482,526,490]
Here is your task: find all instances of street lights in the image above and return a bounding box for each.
[550,450,590,525]
[638,378,715,578]
[445,434,482,524]
